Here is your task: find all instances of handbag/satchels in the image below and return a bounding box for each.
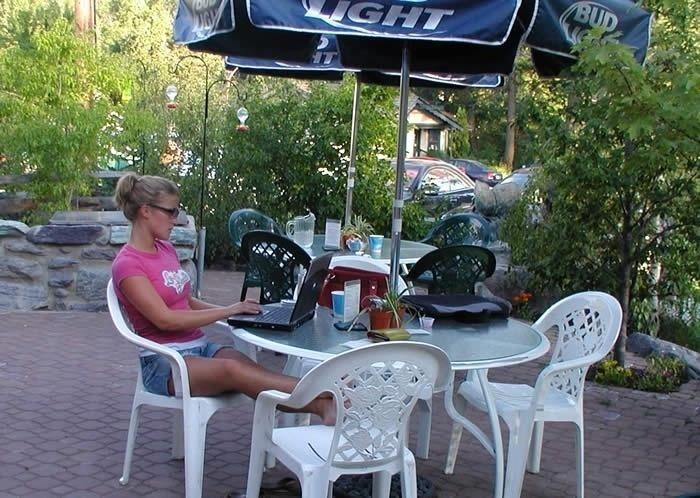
[317,266,390,310]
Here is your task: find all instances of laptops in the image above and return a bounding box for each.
[227,251,333,332]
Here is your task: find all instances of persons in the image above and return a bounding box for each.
[112,170,348,430]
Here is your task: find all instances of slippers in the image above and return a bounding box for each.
[228,477,348,498]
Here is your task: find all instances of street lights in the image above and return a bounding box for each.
[167,56,248,229]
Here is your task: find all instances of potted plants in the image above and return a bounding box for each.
[343,216,375,252]
[361,277,419,329]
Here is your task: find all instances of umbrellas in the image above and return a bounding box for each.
[214,35,507,245]
[173,0,654,314]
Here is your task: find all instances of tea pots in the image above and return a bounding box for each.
[285,212,315,248]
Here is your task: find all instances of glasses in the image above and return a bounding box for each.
[148,203,179,217]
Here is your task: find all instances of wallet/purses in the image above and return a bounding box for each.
[367,328,411,342]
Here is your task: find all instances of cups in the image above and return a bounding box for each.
[368,234,384,259]
[419,317,435,330]
[331,290,345,319]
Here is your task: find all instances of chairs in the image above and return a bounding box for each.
[247,341,453,498]
[107,277,276,498]
[402,244,497,296]
[444,291,623,498]
[229,209,285,248]
[294,255,410,304]
[418,214,497,247]
[240,231,312,304]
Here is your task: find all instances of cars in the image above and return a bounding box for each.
[388,156,547,224]
